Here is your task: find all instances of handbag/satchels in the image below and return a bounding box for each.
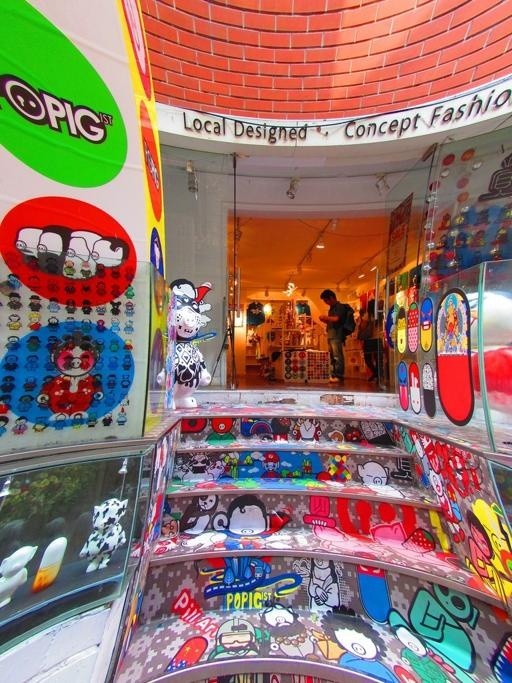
[356,310,375,340]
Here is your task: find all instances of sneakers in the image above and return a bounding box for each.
[329,376,345,384]
[367,371,378,383]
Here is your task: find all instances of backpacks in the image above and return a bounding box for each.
[341,303,357,337]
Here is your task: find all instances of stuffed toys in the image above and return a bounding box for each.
[158,293,212,409]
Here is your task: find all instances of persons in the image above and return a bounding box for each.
[319,289,354,382]
[356,298,386,383]
